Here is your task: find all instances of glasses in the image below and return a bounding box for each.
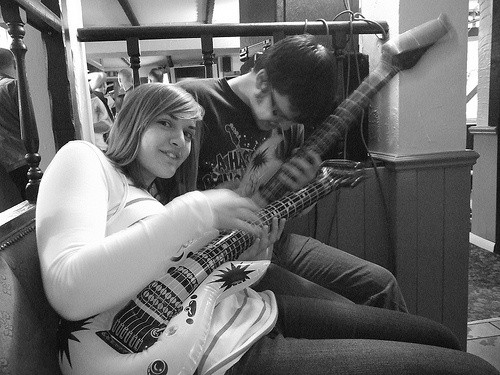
[268,86,302,125]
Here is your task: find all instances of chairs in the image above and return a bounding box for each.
[0,206,60,375]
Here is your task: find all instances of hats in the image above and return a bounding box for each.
[89,71,106,90]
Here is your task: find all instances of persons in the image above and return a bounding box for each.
[117,69,134,91]
[34,81,500,375]
[0,46,29,202]
[89,73,113,152]
[156,32,410,313]
[146,68,164,83]
